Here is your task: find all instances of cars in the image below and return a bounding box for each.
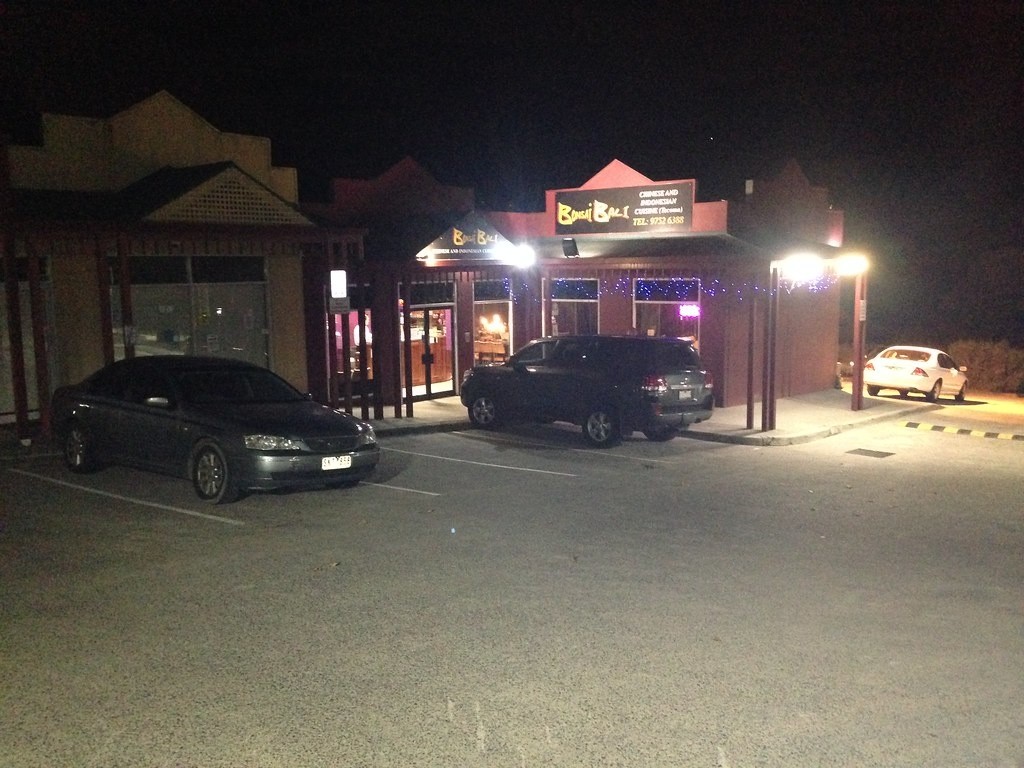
[49,356,379,506]
[863,344,969,402]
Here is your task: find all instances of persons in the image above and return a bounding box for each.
[353,314,373,346]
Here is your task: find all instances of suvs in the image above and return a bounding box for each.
[460,334,717,448]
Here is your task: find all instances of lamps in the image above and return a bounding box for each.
[562,238,580,259]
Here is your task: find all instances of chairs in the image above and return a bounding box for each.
[474,341,506,363]
